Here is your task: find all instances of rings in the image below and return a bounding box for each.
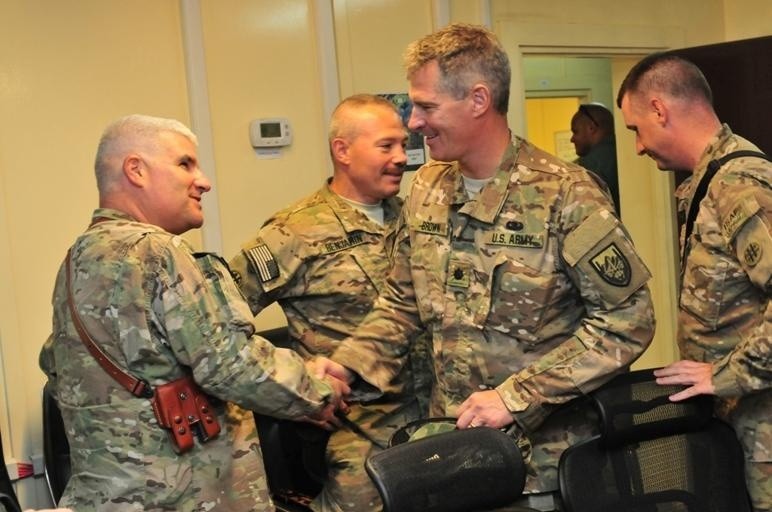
[470,422,476,428]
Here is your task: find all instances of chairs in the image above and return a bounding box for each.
[366,427,538,511]
[557,367,754,510]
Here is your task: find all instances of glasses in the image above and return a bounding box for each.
[579,104,600,126]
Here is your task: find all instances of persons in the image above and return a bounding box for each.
[314,21,656,512]
[226,95,433,512]
[617,52,772,512]
[40,115,351,512]
[570,105,617,217]
